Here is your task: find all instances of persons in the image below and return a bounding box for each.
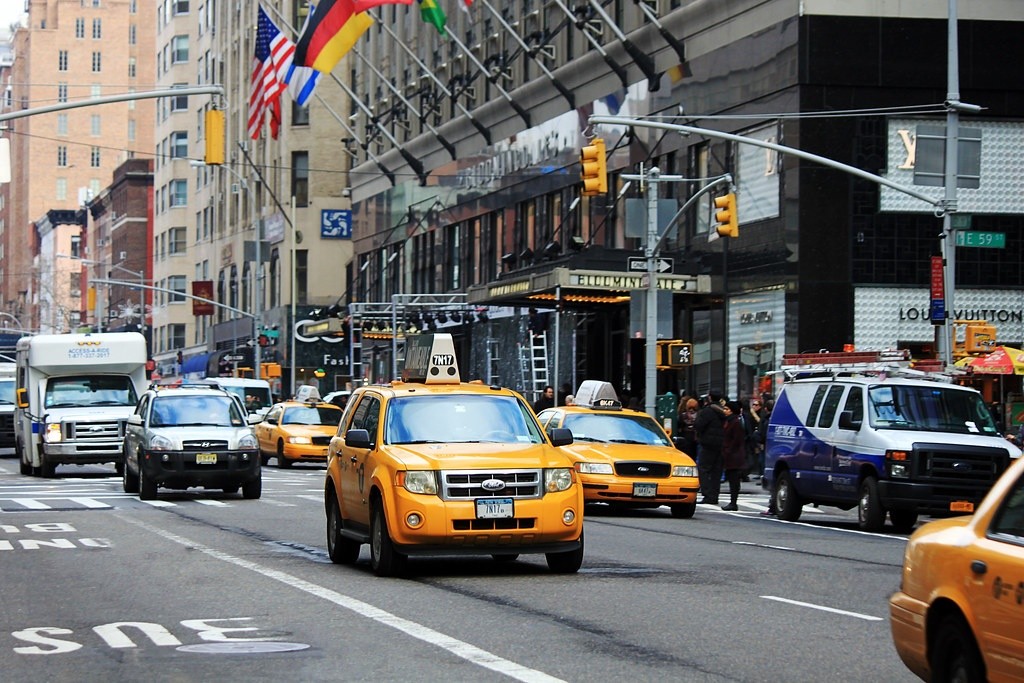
[533,385,576,417]
[989,400,1024,452]
[245,393,283,414]
[332,396,346,407]
[618,388,775,510]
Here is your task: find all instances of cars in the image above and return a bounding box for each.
[535,380,699,518]
[325,390,351,407]
[255,384,345,468]
[887,452,1024,683]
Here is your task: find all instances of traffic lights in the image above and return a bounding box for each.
[713,195,739,237]
[581,139,606,194]
[669,344,694,367]
[241,369,254,379]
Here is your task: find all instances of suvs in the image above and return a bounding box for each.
[325,333,585,575]
[121,384,262,499]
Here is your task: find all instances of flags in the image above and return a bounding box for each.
[247,3,289,140]
[282,0,473,108]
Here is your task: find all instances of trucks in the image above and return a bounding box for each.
[11,333,148,477]
[0,328,34,455]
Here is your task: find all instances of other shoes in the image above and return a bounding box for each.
[760,511,778,518]
[697,498,718,505]
[722,503,738,511]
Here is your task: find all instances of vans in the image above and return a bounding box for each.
[207,377,273,416]
[763,365,1024,533]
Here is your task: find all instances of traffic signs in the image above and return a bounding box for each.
[956,233,1004,249]
[243,337,276,346]
[627,257,673,275]
[222,354,245,362]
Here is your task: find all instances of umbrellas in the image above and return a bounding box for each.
[952,344,1024,437]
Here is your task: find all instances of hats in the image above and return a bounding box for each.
[725,401,741,415]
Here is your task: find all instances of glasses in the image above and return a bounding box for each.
[753,404,759,406]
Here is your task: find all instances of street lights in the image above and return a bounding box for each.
[55,254,102,333]
[80,258,144,334]
[190,160,260,380]
